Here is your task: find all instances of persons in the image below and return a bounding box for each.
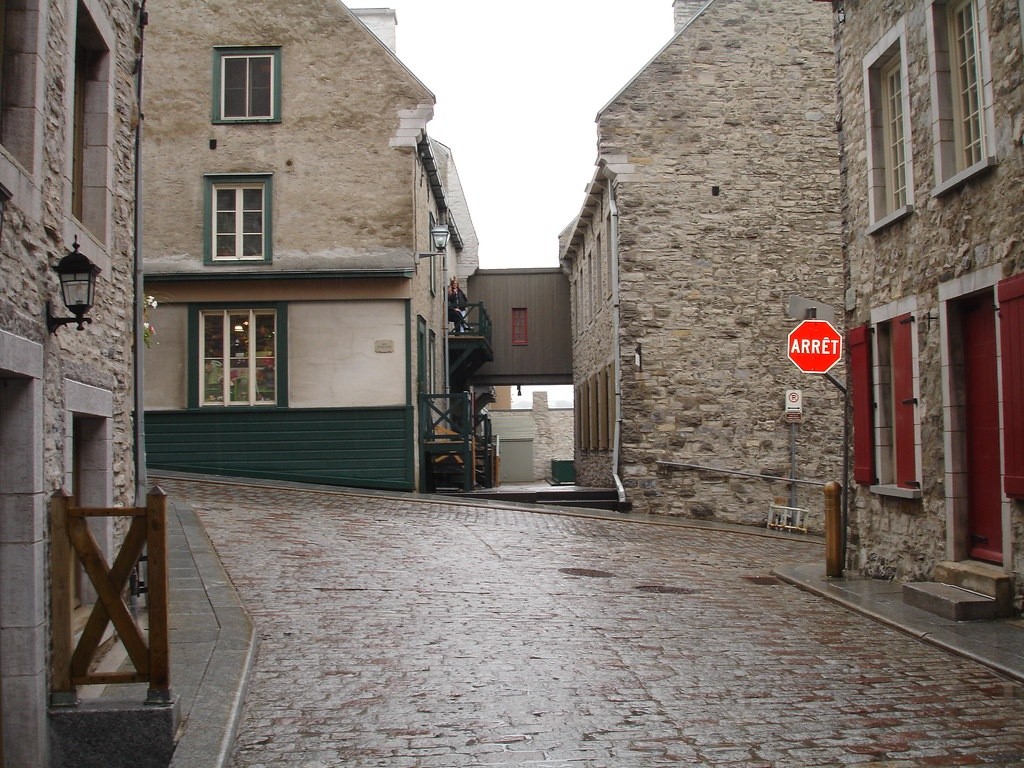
[446,286,474,335]
[450,278,468,333]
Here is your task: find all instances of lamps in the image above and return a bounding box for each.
[45,235,104,337]
[419,224,450,258]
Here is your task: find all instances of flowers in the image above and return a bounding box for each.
[142,289,174,350]
